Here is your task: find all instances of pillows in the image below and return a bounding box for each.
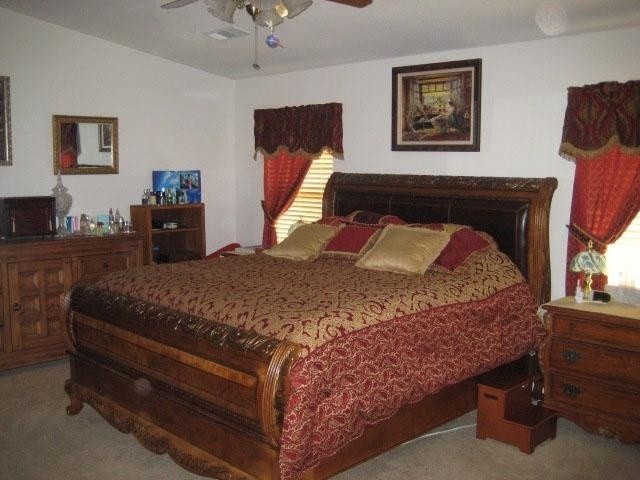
[262,209,489,276]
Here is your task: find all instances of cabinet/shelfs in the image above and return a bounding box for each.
[131,202,206,262]
[0,234,144,371]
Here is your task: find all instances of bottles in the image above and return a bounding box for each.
[140,189,188,205]
[80,208,133,234]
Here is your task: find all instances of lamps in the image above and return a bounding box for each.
[569,239,607,303]
[208,1,313,48]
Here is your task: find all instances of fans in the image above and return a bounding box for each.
[161,0,373,49]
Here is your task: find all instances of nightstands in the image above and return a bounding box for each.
[542,296,640,443]
[221,246,262,257]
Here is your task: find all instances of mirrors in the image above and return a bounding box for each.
[52,116,118,174]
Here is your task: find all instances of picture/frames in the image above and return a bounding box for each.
[0,76,12,167]
[389,59,482,152]
[98,122,112,152]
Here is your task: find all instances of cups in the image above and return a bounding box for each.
[167,222,177,229]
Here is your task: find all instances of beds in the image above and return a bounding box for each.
[65,173,557,480]
